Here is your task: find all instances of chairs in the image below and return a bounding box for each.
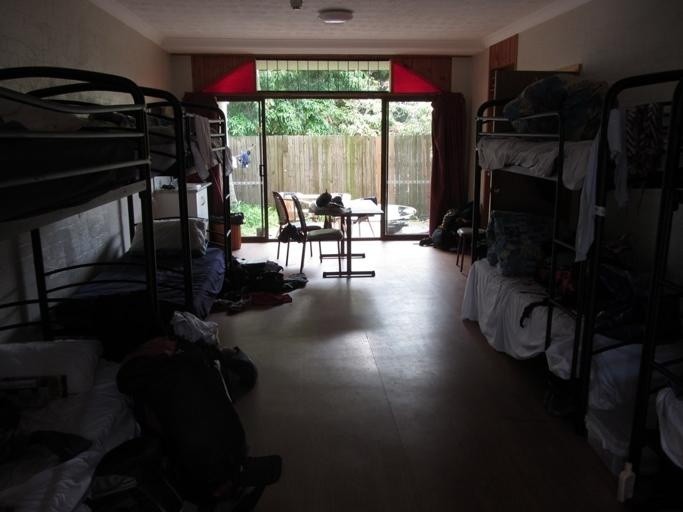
[291,195,344,277]
[273,191,321,266]
[352,197,378,238]
[456,227,489,273]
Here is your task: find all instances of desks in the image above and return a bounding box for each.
[314,199,384,278]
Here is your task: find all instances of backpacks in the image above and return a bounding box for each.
[116,350,283,510]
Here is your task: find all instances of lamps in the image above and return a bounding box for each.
[317,9,354,24]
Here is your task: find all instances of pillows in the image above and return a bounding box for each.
[127,218,205,257]
[0,339,99,394]
[490,208,572,278]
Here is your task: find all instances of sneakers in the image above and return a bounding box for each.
[212,299,232,311]
[228,299,252,312]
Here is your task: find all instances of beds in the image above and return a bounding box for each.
[25,82,230,340]
[628,70,683,505]
[0,65,161,512]
[468,72,683,421]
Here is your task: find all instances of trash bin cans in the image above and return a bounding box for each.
[211,212,244,251]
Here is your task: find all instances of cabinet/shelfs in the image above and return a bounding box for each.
[151,180,213,244]
